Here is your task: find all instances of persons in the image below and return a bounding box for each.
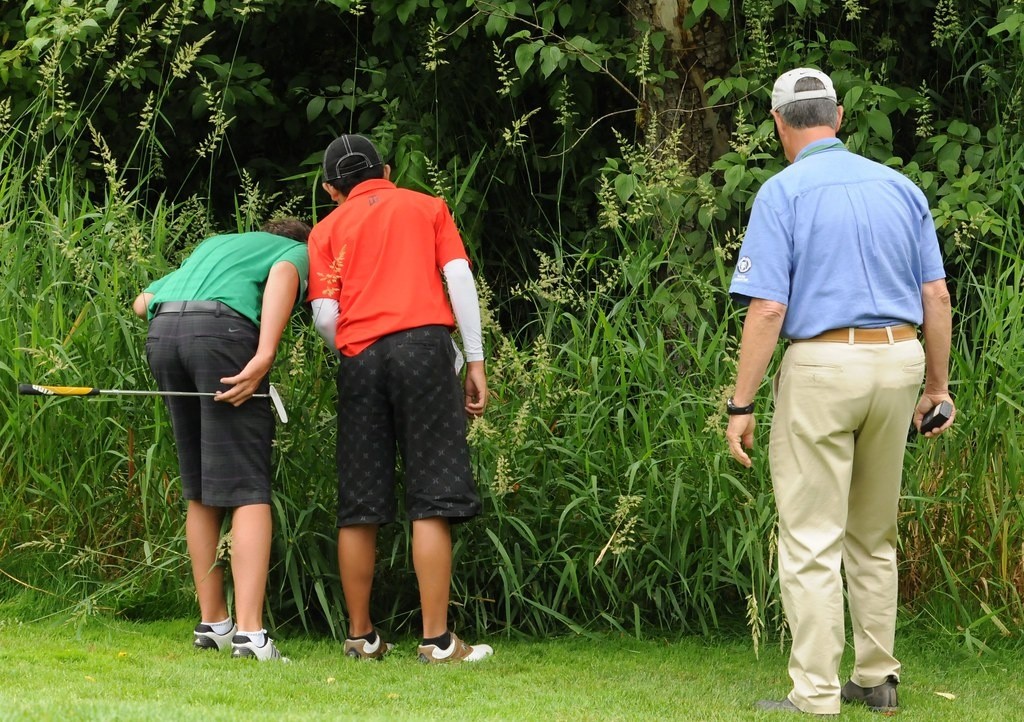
[308,133,494,663]
[725,67,957,715]
[134,219,312,663]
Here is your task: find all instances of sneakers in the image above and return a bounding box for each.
[416,632,494,667]
[192,623,238,653]
[756,697,804,714]
[343,630,396,662]
[841,674,899,711]
[230,633,293,666]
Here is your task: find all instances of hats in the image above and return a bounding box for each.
[771,67,837,110]
[324,134,383,182]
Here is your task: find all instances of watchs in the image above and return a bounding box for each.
[727,396,755,415]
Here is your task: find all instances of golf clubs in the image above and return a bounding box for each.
[18,383,291,428]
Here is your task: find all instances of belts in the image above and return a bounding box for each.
[149,299,247,320]
[790,323,917,344]
